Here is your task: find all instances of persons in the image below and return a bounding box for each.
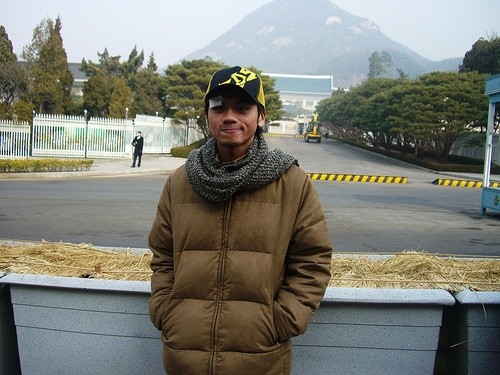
[148,65,333,375]
[129,131,144,167]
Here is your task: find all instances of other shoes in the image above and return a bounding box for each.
[131,165,135,167]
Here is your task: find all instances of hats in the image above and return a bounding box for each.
[137,131,141,134]
[204,66,266,114]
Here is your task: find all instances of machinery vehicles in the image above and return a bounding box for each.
[303,111,323,143]
